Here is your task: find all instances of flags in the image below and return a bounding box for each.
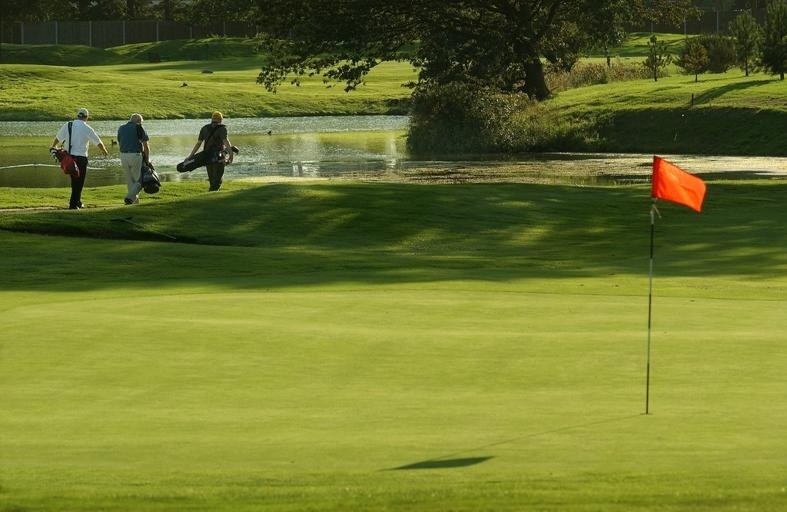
[652,155,707,213]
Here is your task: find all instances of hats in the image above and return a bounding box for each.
[211,111,224,120]
[77,108,89,117]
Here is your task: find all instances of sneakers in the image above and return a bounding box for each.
[69,202,85,209]
[124,198,133,205]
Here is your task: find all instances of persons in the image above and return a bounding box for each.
[50,107,110,210]
[180,109,234,194]
[116,112,151,205]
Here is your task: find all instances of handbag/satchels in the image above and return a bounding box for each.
[175,150,207,174]
[140,158,160,194]
[56,148,80,178]
